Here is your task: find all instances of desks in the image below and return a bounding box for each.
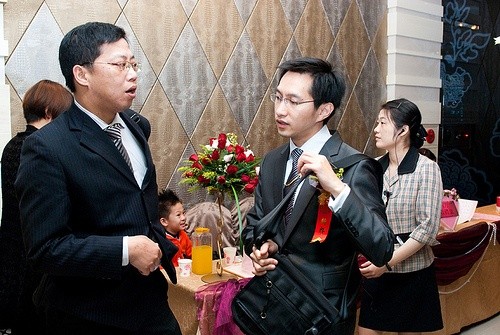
[160,204,500,335]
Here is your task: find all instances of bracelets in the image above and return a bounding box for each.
[385,262,392,272]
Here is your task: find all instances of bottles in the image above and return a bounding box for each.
[192,228,212,274]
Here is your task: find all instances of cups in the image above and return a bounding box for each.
[178,259,192,277]
[223,247,236,265]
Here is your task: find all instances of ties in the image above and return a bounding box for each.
[282,148,304,228]
[102,123,133,175]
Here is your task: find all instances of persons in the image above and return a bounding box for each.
[359,98,444,335]
[230,57,394,335]
[14,23,182,335]
[0,80,84,335]
[157,188,193,270]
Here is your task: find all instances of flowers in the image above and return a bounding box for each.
[309,168,344,207]
[177,132,262,257]
[443,188,459,203]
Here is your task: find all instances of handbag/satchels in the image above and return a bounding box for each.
[231,251,347,335]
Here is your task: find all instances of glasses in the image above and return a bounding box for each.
[270,94,322,106]
[80,61,139,73]
